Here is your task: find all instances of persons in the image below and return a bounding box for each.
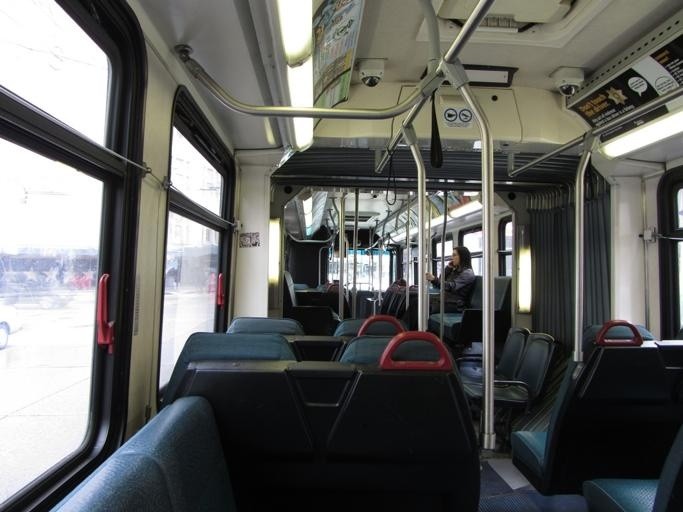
[403,246,476,327]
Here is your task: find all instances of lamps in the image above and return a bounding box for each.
[253,1,316,155]
[295,193,317,239]
[517,221,534,318]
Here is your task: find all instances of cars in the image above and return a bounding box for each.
[0,277,74,351]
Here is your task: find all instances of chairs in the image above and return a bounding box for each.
[42,271,681,512]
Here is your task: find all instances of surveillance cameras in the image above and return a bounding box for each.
[552,67,585,97]
[371,193,379,198]
[358,58,385,87]
